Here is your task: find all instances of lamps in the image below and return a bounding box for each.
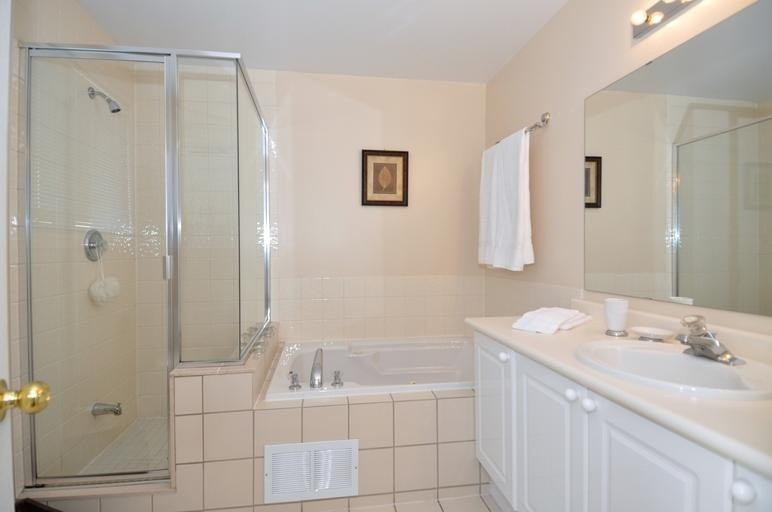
[628,8,651,26]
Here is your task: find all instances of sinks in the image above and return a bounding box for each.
[574,338,772,400]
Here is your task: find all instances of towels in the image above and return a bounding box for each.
[477,127,535,272]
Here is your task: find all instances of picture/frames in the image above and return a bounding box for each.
[361,150,408,206]
[585,157,601,209]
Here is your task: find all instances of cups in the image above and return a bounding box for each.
[605,297,629,331]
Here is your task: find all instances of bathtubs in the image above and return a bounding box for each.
[263,334,475,401]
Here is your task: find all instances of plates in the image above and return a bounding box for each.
[631,326,675,340]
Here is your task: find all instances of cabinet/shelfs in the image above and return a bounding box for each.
[473,331,772,512]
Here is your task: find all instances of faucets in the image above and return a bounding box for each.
[308,347,323,388]
[675,314,749,367]
[90,400,122,416]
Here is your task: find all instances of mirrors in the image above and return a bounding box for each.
[583,0,772,316]
